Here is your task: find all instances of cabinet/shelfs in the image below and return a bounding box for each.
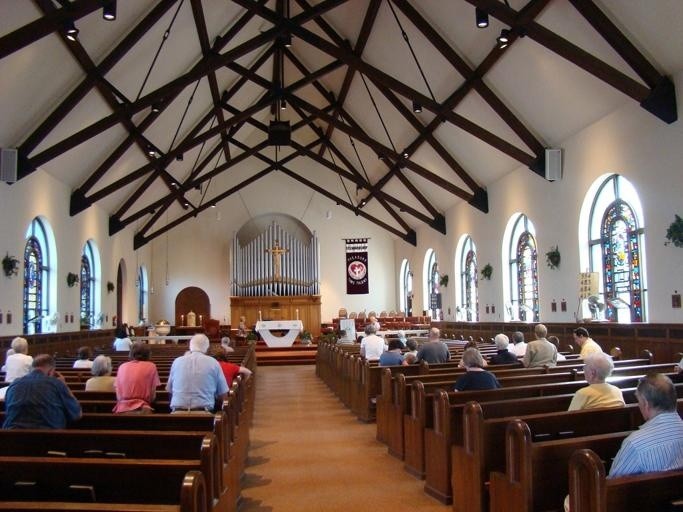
[431,322,683,357]
[0,329,118,368]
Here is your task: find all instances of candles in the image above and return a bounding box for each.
[296,309,299,319]
[200,315,203,325]
[259,311,262,321]
[182,314,184,325]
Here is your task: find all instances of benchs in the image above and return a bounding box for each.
[77,401,228,494]
[569,449,683,512]
[52,345,256,491]
[489,418,639,512]
[388,356,679,460]
[425,383,683,504]
[316,333,683,421]
[0,457,213,512]
[0,430,233,511]
[0,413,232,510]
[452,398,683,512]
[404,366,682,480]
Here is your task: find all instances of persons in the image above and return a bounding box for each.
[113,328,133,351]
[2,354,82,429]
[402,339,418,365]
[379,339,408,366]
[490,333,518,365]
[523,324,558,369]
[239,316,246,335]
[360,325,387,366]
[337,330,351,346]
[5,338,32,382]
[207,343,250,399]
[416,328,451,363]
[607,372,682,477]
[573,329,601,363]
[85,355,116,393]
[222,336,234,352]
[365,316,380,332]
[511,332,528,357]
[454,347,502,392]
[112,341,162,414]
[458,341,488,368]
[569,352,625,412]
[166,335,229,414]
[547,336,566,362]
[72,346,94,368]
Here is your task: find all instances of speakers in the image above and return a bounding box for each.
[545,149,561,180]
[2,149,18,182]
